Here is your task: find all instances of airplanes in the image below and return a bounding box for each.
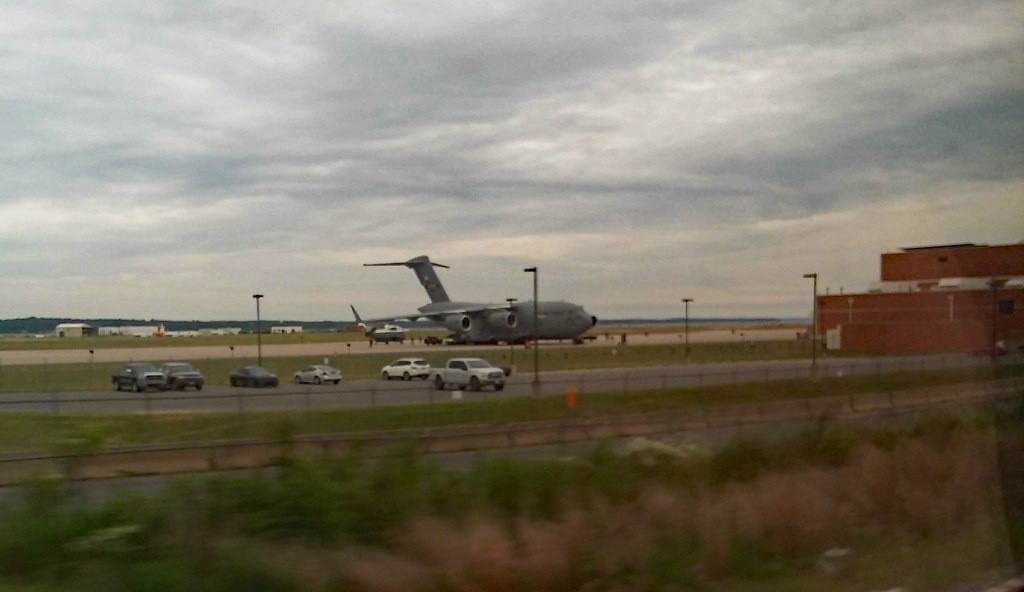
[362,254,598,346]
[349,304,411,346]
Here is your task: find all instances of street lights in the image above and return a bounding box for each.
[252,293,264,367]
[524,266,540,388]
[681,298,694,354]
[803,273,818,376]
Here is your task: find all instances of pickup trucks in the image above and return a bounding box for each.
[427,357,506,392]
[111,362,168,393]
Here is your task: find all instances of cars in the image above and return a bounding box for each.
[293,364,342,385]
[380,358,430,381]
[230,366,278,388]
[160,362,204,390]
